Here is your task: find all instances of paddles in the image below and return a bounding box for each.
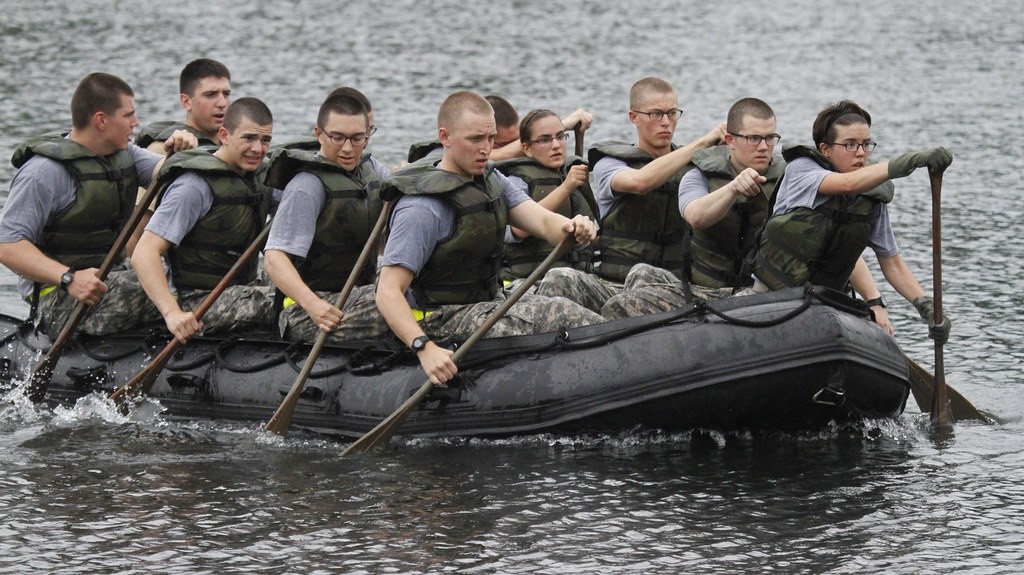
[743,184,989,428]
[572,122,586,159]
[22,143,178,405]
[338,221,592,458]
[107,216,274,416]
[265,200,395,438]
[927,164,957,425]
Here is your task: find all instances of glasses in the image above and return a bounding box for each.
[366,127,377,136]
[632,108,683,122]
[319,126,368,146]
[727,132,781,146]
[826,141,877,153]
[526,134,569,145]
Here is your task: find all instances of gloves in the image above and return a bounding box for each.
[914,296,951,345]
[887,147,953,178]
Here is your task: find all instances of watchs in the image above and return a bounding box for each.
[60,267,76,289]
[411,336,430,353]
[868,296,887,307]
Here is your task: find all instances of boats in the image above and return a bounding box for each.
[1,286,912,442]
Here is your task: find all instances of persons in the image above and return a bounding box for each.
[734,100,953,345]
[1,72,198,336]
[537,77,729,311]
[374,92,604,387]
[603,97,895,338]
[503,107,601,292]
[135,58,233,211]
[131,98,283,345]
[325,87,378,148]
[264,95,391,345]
[423,96,594,157]
